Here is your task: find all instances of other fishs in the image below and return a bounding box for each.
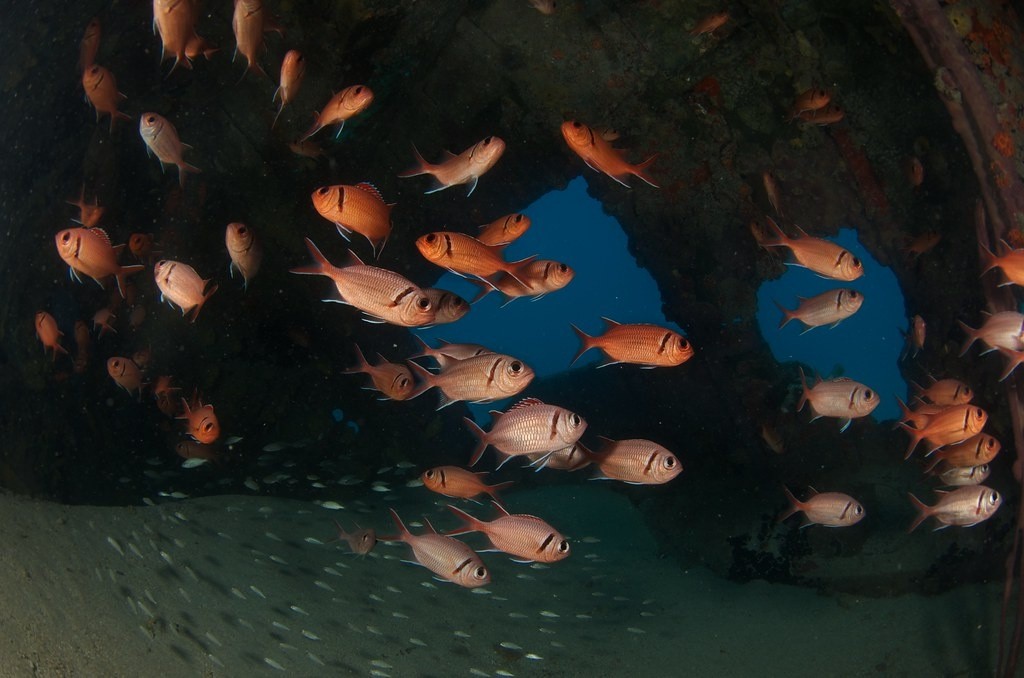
[34,0,1024,587]
